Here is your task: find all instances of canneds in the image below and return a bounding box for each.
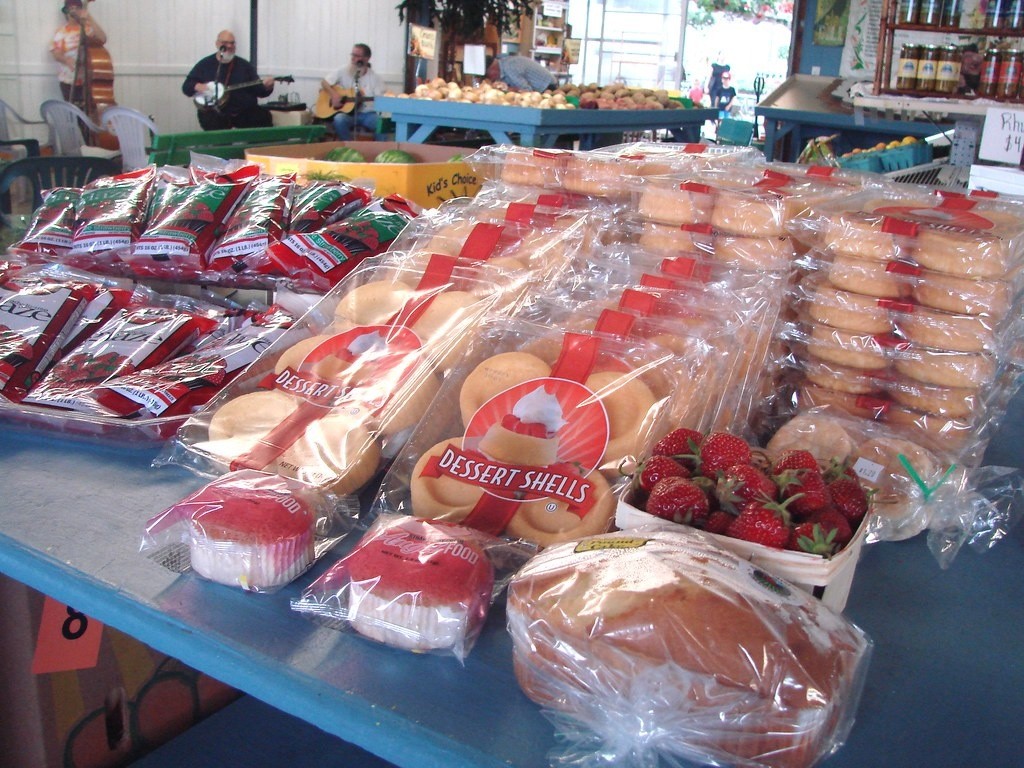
[895,0,1024,99]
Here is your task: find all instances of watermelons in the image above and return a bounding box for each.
[323,146,418,163]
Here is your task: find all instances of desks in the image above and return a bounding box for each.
[372,96,719,150]
[0,386,1024,768]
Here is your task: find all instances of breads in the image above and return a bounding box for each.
[763,200,1023,543]
[206,202,706,556]
[509,530,867,768]
[451,144,866,440]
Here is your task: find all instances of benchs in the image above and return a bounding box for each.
[148,124,327,168]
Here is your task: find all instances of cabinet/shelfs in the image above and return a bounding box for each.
[519,0,569,74]
[872,0,1024,105]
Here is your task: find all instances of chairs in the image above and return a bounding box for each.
[715,119,758,146]
[102,108,158,176]
[38,100,121,188]
[0,156,121,214]
[0,99,51,203]
[0,139,41,213]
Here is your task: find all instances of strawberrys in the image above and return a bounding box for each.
[629,427,870,562]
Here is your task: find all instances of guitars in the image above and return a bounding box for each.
[194,74,295,110]
[314,84,374,118]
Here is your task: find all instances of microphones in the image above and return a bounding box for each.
[357,60,364,69]
[219,46,227,58]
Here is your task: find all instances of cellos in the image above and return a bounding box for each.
[79,0,121,151]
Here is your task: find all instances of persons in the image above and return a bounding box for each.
[705,49,730,130]
[481,54,558,91]
[671,52,686,82]
[321,43,387,142]
[50,0,108,145]
[182,30,274,146]
[687,80,703,103]
[714,72,737,133]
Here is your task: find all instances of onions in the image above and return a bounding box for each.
[381,77,575,109]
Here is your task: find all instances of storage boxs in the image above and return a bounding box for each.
[1,571,246,768]
[837,142,937,173]
[242,136,504,209]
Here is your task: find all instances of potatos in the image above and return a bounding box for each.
[544,81,686,110]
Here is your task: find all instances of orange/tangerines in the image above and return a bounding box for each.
[841,136,917,157]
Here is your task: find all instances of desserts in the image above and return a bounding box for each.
[349,522,496,649]
[186,483,315,589]
